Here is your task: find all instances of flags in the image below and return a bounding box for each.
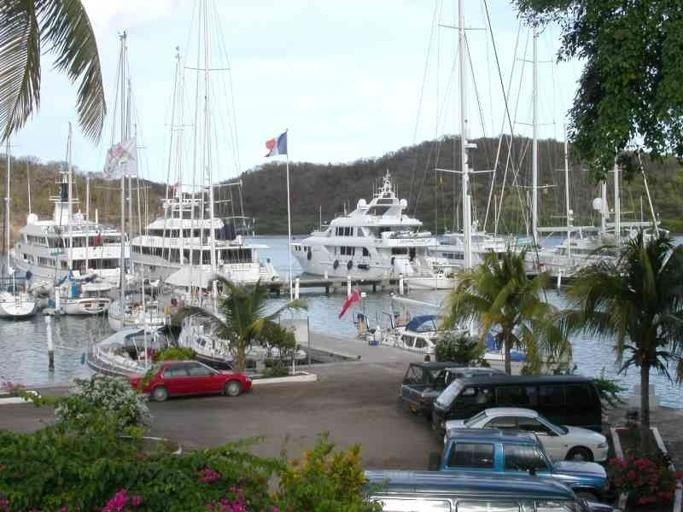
[338,287,360,319]
[263,132,287,157]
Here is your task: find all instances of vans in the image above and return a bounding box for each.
[431,376,603,437]
[364,468,622,512]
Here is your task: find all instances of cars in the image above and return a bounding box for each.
[130,357,253,404]
[442,406,610,466]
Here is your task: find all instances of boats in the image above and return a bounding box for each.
[429,2,672,283]
[0,276,303,360]
[367,315,526,361]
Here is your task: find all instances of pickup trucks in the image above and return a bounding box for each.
[398,362,481,423]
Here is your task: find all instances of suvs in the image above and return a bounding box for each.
[437,428,609,501]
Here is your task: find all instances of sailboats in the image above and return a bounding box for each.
[132,0,267,288]
[13,120,130,295]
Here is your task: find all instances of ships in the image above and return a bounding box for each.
[289,170,455,287]
